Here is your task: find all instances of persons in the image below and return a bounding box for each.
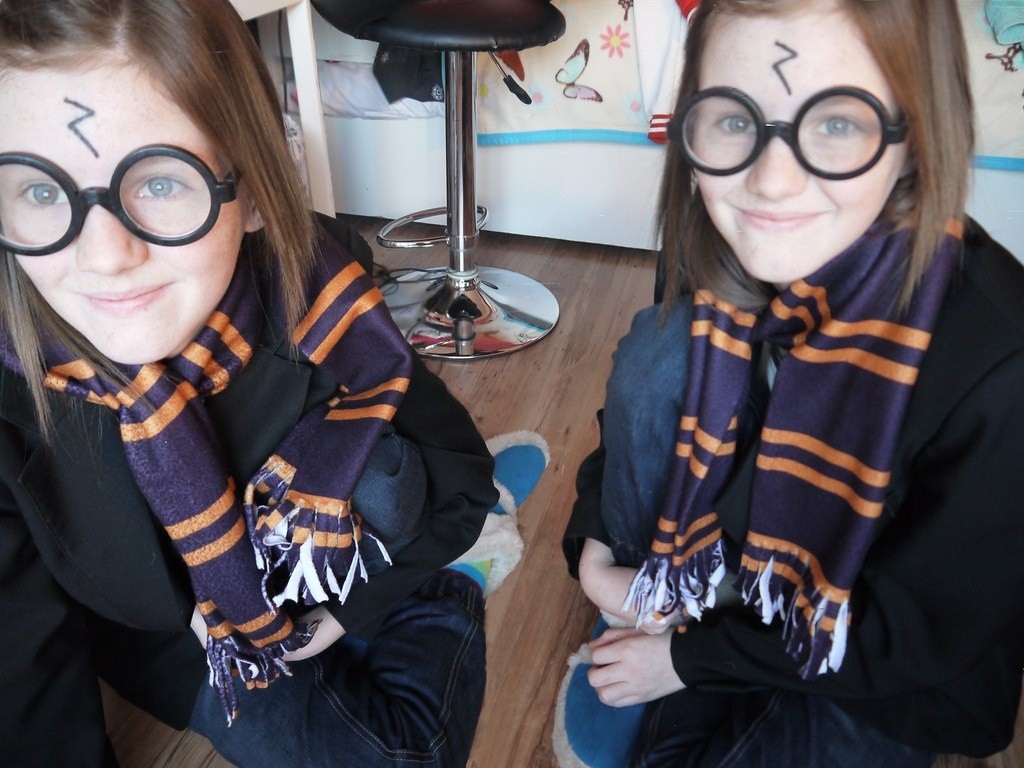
[0,0,548,768]
[551,0,1024,768]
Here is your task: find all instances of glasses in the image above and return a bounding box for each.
[666,86,912,177]
[0,145,242,256]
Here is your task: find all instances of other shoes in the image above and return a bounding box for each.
[552,605,645,768]
[445,429,551,601]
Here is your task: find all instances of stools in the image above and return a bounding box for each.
[308,0,567,361]
